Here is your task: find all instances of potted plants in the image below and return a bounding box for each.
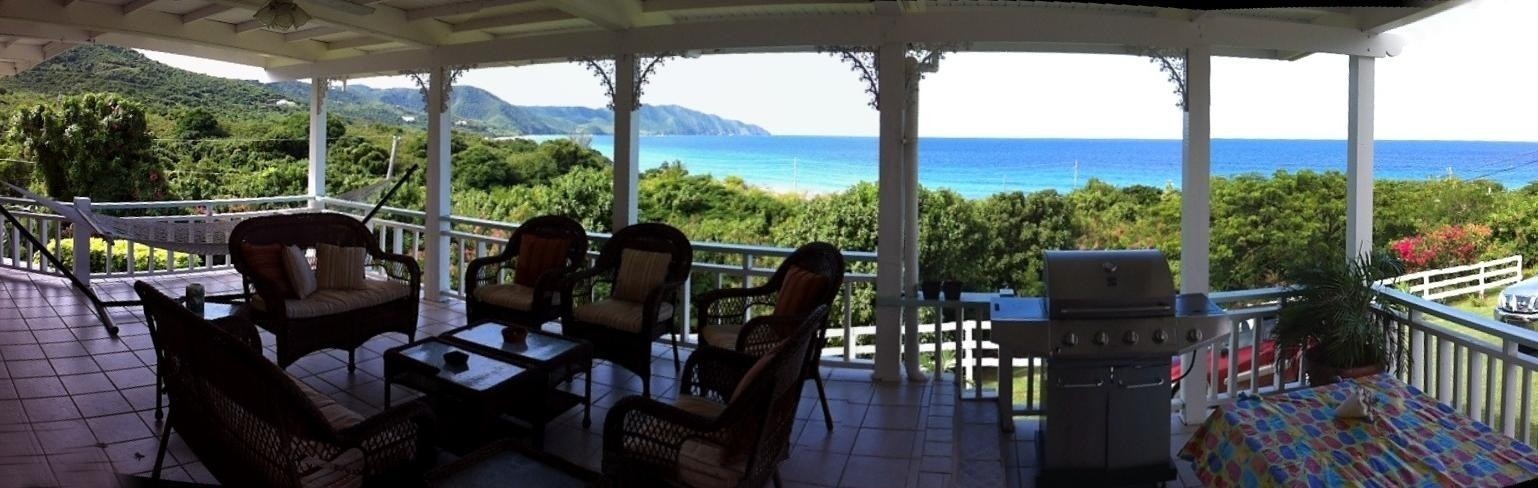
[921,272,942,298]
[943,280,961,300]
[1262,239,1415,388]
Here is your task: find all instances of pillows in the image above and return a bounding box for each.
[612,248,672,303]
[281,243,318,300]
[239,241,299,299]
[315,243,367,289]
[773,261,827,314]
[719,335,792,466]
[517,234,577,287]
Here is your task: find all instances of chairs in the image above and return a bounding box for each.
[682,242,845,431]
[601,304,834,487]
[559,223,693,400]
[465,215,588,339]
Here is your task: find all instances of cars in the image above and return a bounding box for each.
[1498,276,1538,342]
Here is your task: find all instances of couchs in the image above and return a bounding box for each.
[228,213,421,374]
[133,280,437,488]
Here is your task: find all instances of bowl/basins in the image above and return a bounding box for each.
[501,325,528,342]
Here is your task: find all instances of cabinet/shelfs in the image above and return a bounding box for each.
[1046,360,1170,467]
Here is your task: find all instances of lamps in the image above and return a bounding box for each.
[253,1,312,31]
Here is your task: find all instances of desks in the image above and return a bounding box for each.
[423,436,606,488]
[383,336,528,457]
[1175,372,1538,488]
[437,318,593,429]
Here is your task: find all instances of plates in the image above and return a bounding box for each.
[444,351,470,368]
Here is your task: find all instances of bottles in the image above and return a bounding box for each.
[186,283,204,316]
